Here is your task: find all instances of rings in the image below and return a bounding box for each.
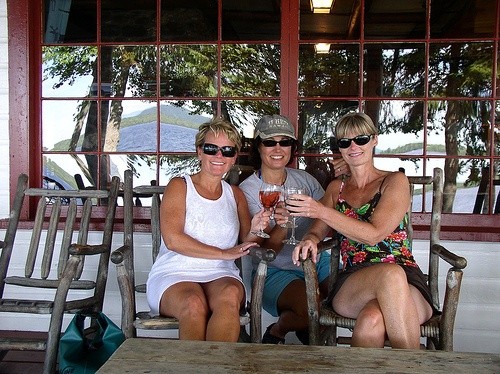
[338,168,340,171]
[240,248,242,251]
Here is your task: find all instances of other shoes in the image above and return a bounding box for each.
[294,329,311,345]
[262,322,285,344]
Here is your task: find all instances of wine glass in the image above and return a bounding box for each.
[249,183,281,238]
[277,185,299,228]
[282,187,306,245]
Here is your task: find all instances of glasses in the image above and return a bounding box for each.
[201,143,237,158]
[337,134,372,149]
[260,138,294,147]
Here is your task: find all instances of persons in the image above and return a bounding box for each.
[293,114,435,348]
[145,120,271,344]
[238,113,351,343]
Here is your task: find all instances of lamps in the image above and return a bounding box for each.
[314,43,331,53]
[309,0,335,13]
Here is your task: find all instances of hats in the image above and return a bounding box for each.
[254,115,297,140]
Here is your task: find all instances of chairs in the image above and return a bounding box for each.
[0,174,120,374]
[112,168,276,344]
[298,168,467,353]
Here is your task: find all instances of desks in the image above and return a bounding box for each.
[95,338,500,374]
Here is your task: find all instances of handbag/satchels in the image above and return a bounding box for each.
[57,307,126,374]
[312,158,333,191]
